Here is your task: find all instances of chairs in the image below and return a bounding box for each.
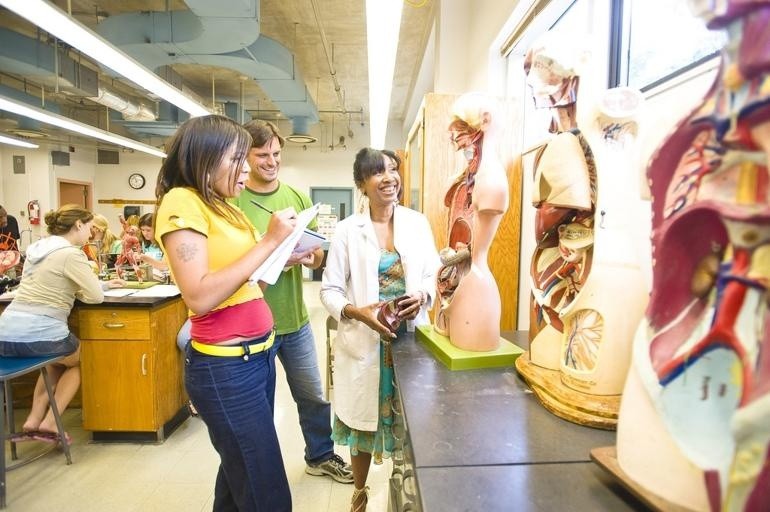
[324,315,340,401]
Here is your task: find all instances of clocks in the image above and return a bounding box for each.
[128,173,145,189]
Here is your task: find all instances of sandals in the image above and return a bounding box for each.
[350,486,370,511]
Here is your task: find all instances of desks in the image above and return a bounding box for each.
[413,462,651,512]
[388,329,645,468]
[0,284,199,445]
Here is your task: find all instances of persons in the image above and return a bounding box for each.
[139,212,162,266]
[0,204,105,446]
[0,205,20,249]
[88,215,115,252]
[321,147,441,512]
[228,118,353,483]
[155,115,299,512]
[114,213,143,285]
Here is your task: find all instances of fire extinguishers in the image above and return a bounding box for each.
[27,200,40,224]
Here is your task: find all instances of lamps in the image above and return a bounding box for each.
[0,134,40,151]
[0,96,169,159]
[0,0,210,117]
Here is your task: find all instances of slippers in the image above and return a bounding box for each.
[9,435,73,448]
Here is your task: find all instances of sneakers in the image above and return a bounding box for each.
[304,453,355,484]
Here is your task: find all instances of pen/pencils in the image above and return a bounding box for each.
[250,200,273,214]
[126,290,139,296]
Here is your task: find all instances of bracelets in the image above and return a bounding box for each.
[342,303,351,320]
[417,289,427,305]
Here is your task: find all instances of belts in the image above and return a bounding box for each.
[191,331,277,357]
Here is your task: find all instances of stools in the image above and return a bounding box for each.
[0,353,73,509]
